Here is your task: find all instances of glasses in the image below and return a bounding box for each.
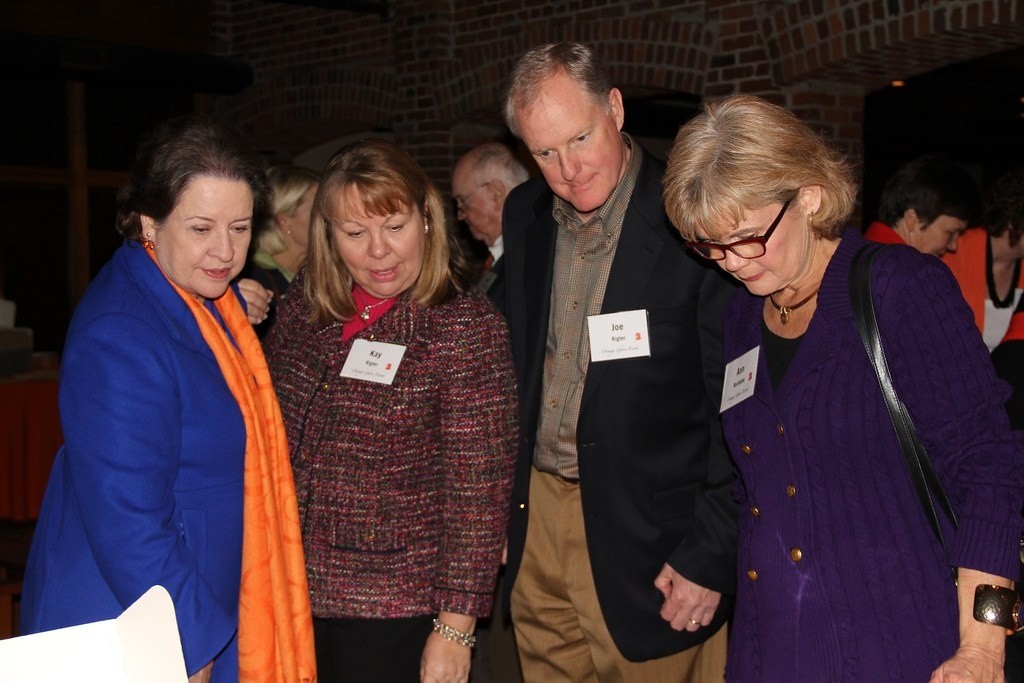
[686,195,796,261]
[456,182,490,213]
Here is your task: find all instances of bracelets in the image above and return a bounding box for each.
[432,619,477,647]
[972,584,1021,635]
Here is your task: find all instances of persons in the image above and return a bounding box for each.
[450,142,531,313]
[231,162,321,342]
[237,138,520,683]
[18,112,318,683]
[862,154,1024,354]
[486,42,737,683]
[660,93,1024,683]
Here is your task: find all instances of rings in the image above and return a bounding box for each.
[689,618,700,625]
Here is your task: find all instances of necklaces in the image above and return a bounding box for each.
[769,289,819,325]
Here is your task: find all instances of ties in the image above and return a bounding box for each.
[484,255,495,276]
